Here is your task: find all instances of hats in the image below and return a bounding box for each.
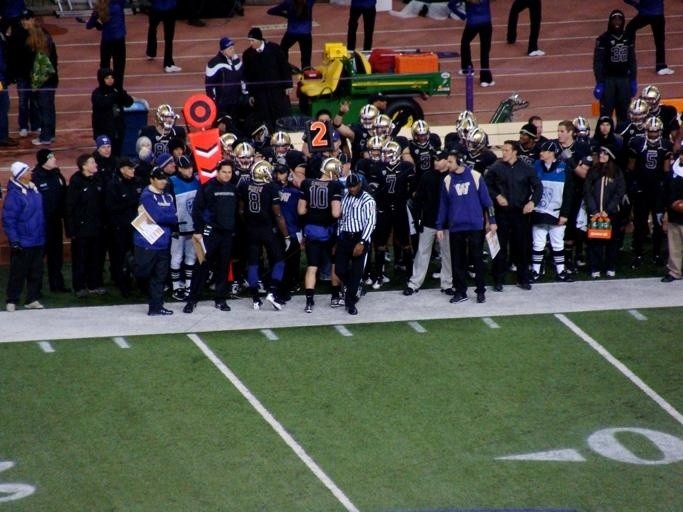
[220,37,235,51]
[612,13,622,16]
[520,124,559,153]
[120,136,194,179]
[600,144,616,159]
[10,162,30,180]
[97,135,112,149]
[320,130,360,187]
[37,149,56,165]
[248,28,262,40]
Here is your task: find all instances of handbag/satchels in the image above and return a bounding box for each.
[587,215,612,240]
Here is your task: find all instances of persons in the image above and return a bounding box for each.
[0,0,683,317]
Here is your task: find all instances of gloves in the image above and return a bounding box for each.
[285,236,291,251]
[630,81,637,97]
[593,83,603,100]
[10,242,22,251]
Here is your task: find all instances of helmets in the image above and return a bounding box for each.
[219,131,291,185]
[360,104,402,165]
[628,85,664,143]
[153,104,180,130]
[412,120,429,141]
[455,111,488,156]
[572,116,590,140]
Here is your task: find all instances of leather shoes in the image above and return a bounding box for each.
[661,274,675,282]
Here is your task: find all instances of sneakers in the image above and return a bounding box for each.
[49,288,106,298]
[458,70,474,75]
[654,256,664,268]
[0,128,55,147]
[657,68,675,76]
[165,65,182,73]
[394,251,615,304]
[24,301,44,309]
[6,303,16,312]
[148,251,391,315]
[632,256,642,269]
[480,80,496,87]
[529,50,545,56]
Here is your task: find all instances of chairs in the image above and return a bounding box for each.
[300,59,344,96]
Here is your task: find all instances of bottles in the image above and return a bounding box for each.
[590,217,611,230]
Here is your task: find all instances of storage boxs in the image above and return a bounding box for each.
[393,53,439,75]
[368,48,400,74]
